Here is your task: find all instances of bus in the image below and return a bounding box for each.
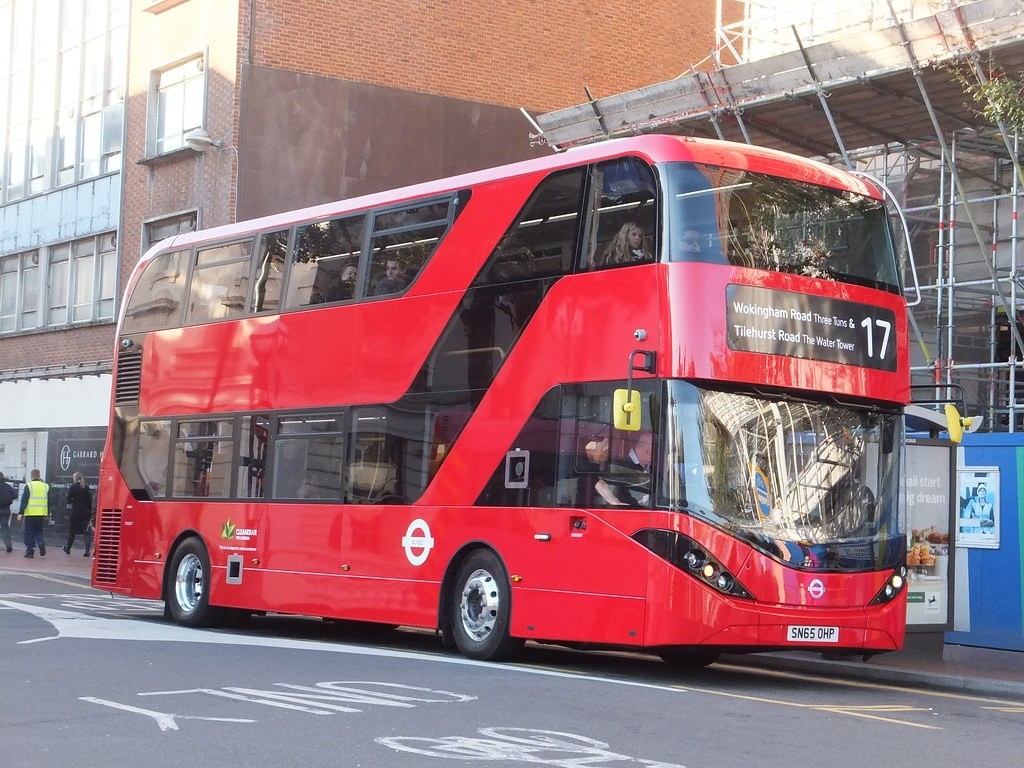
[90,134,974,671]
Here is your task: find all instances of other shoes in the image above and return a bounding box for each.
[23,551,34,558]
[83,550,90,556]
[6,547,12,552]
[40,552,46,556]
[63,546,72,554]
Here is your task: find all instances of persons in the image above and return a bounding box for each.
[374,258,406,296]
[615,433,652,506]
[279,438,322,499]
[602,222,652,265]
[17,469,52,558]
[334,263,358,301]
[62,472,93,557]
[142,430,169,494]
[0,472,18,552]
[971,484,994,534]
[678,227,702,253]
